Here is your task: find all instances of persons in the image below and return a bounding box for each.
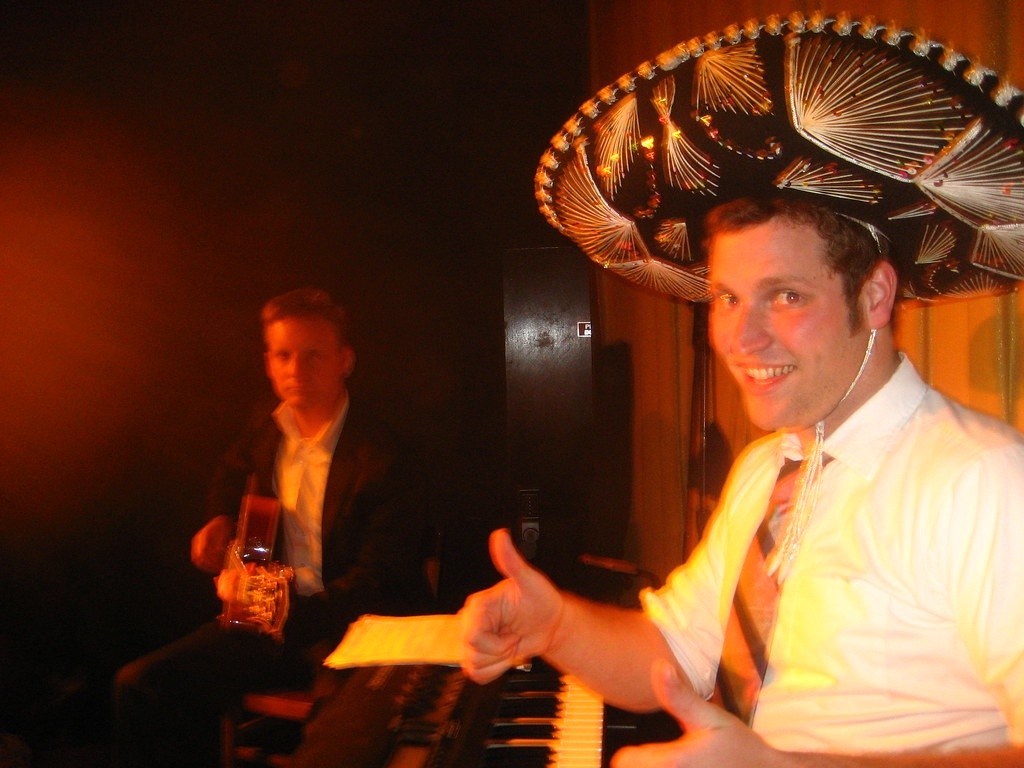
[111,289,437,767]
[459,11,1024,768]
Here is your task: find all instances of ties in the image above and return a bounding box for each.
[707,450,837,726]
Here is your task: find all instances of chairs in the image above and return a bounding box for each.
[213,518,449,768]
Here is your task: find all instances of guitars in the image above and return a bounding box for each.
[214,489,296,639]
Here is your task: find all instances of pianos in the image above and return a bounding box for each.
[290,653,606,768]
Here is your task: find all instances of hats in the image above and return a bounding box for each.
[532,10,1024,302]
